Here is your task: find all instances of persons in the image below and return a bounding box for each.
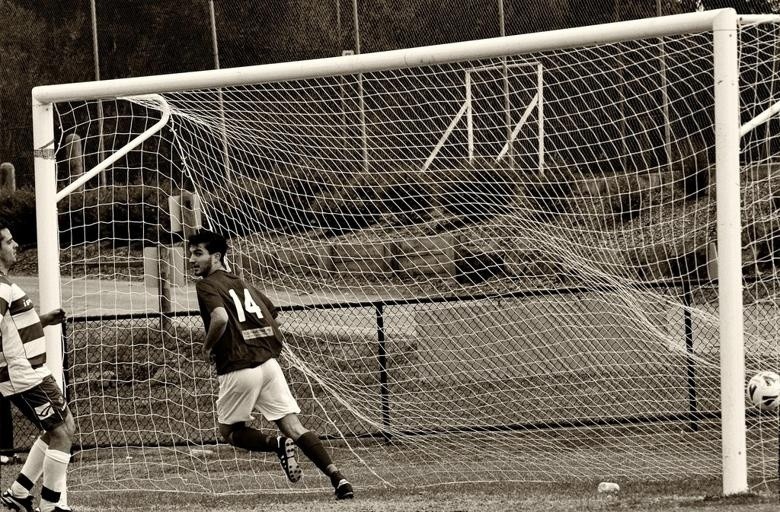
[188,230,354,499]
[0,228,75,511]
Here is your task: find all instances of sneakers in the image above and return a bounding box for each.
[276,435,302,482]
[335,479,354,500]
[35,505,73,512]
[0,487,34,512]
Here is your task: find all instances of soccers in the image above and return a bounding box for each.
[748,370,779,409]
